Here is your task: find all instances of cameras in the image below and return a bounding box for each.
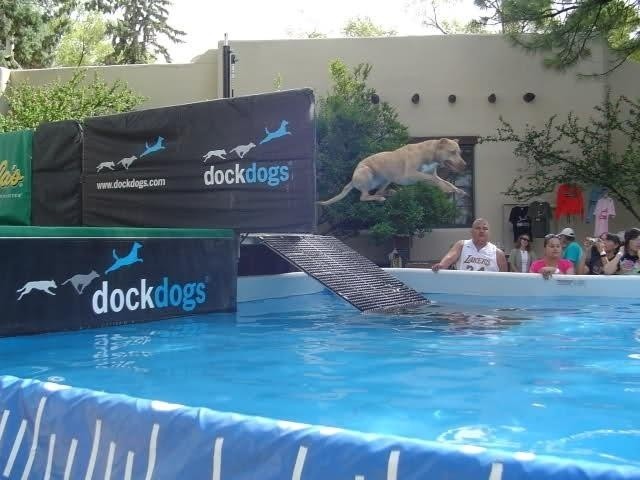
[585,236,599,246]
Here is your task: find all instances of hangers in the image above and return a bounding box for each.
[519,199,543,206]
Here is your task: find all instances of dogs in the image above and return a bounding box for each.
[315,138,468,206]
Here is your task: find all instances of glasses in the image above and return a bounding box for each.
[521,238,530,242]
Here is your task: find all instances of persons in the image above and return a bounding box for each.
[432,218,508,273]
[557,228,583,273]
[529,234,574,280]
[508,235,536,272]
[582,227,640,274]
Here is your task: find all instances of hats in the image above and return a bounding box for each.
[556,228,575,237]
